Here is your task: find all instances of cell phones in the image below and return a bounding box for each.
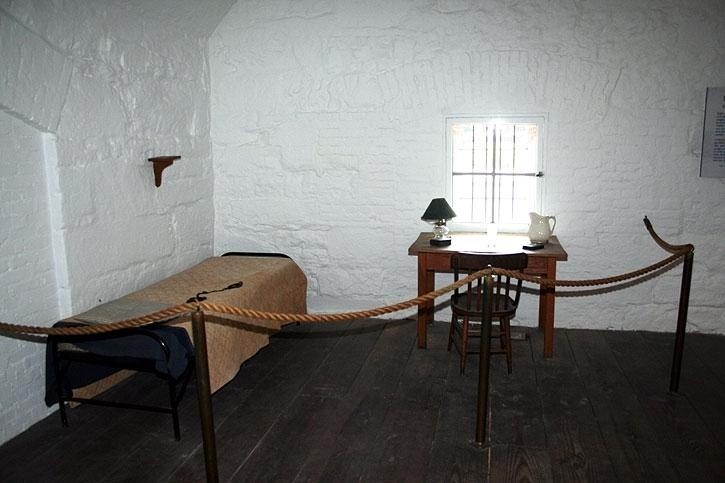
[523,243,544,250]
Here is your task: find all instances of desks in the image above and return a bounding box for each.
[406,231,569,358]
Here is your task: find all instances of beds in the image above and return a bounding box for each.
[44,252,307,442]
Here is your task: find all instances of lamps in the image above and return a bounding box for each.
[421,198,456,246]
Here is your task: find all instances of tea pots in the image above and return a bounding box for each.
[528,211,556,243]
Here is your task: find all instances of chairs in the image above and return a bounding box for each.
[447,253,529,377]
